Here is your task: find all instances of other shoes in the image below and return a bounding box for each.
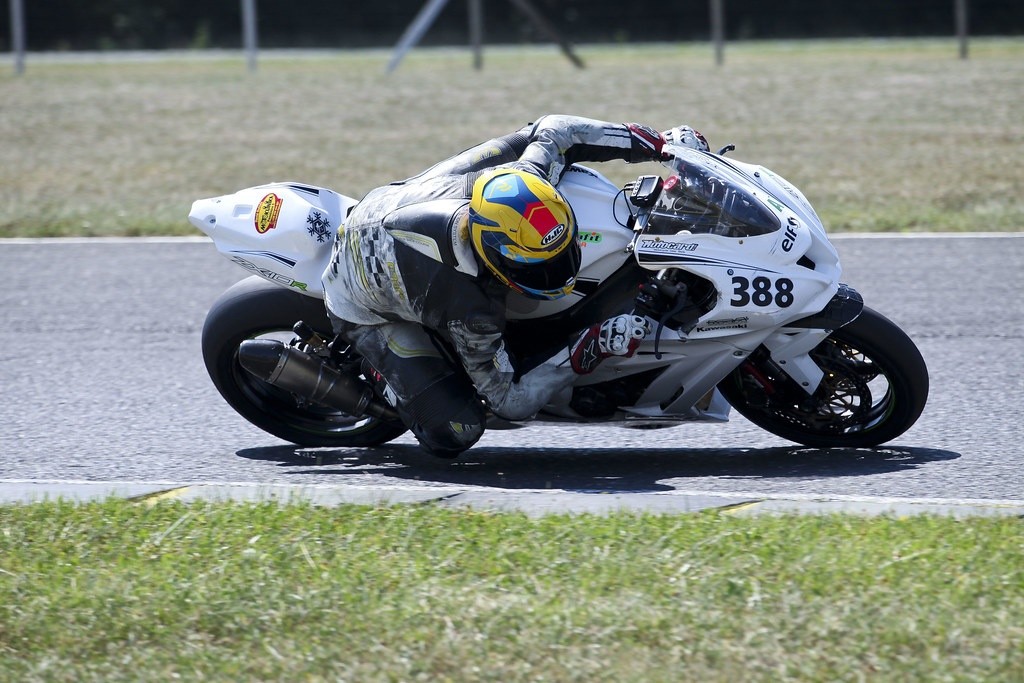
[361,357,397,408]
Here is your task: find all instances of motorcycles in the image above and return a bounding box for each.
[188,141,931,451]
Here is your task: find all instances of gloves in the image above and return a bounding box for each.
[569,314,654,376]
[624,120,710,164]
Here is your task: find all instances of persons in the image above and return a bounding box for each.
[321,115,711,459]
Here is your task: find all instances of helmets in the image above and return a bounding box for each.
[468,169,581,301]
